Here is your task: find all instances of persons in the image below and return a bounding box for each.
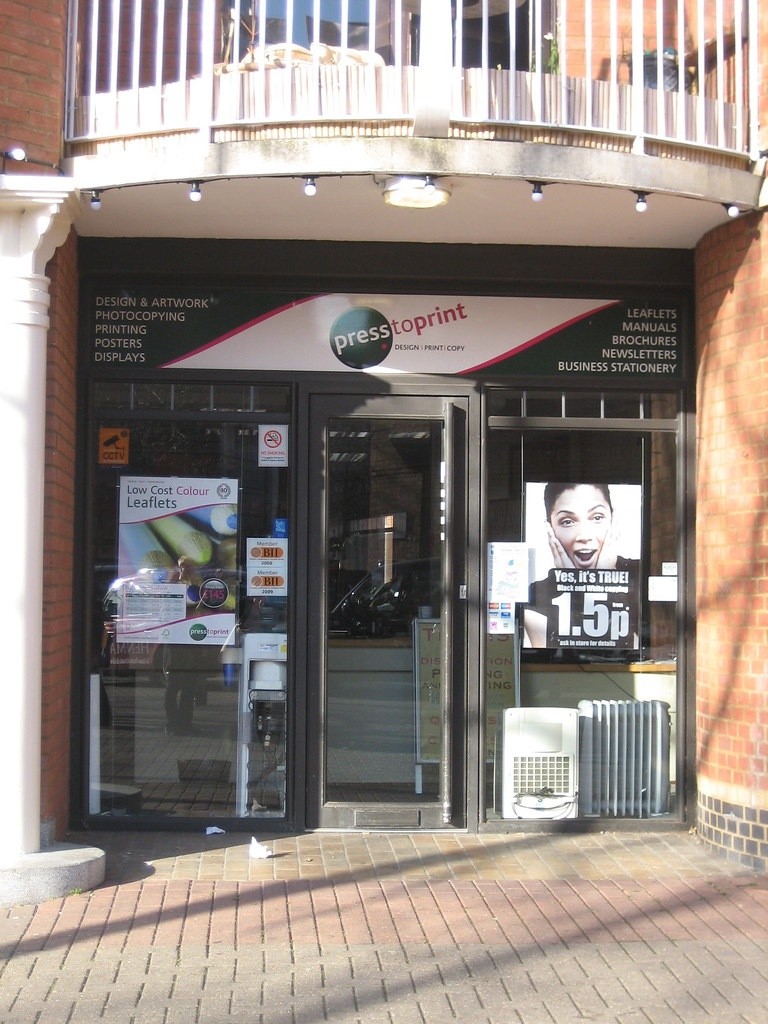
[165,645,201,736]
[524,483,641,648]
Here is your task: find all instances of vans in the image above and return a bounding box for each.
[101,565,244,684]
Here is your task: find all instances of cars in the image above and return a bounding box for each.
[332,556,442,637]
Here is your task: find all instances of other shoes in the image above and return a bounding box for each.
[167,721,203,736]
